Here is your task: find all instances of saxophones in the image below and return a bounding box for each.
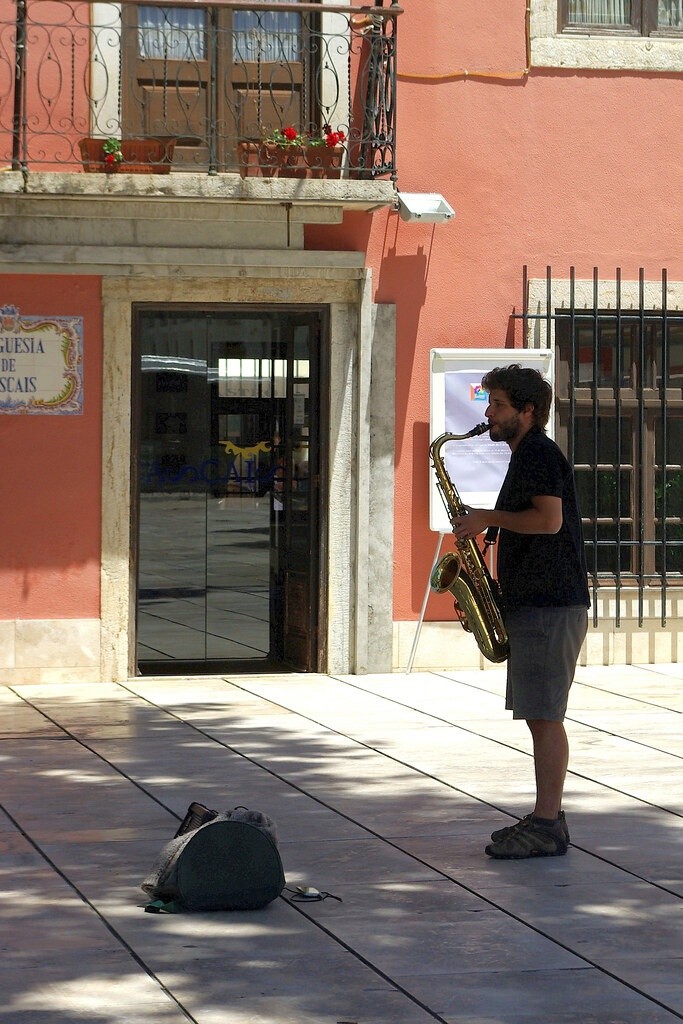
[429,423,510,664]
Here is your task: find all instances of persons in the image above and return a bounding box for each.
[480,364,592,861]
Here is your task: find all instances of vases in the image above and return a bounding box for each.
[78,138,177,175]
[237,143,344,179]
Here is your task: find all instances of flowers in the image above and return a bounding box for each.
[102,136,126,169]
[266,126,346,149]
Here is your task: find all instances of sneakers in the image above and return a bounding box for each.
[485,811,570,859]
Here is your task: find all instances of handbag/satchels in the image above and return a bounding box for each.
[141,806,286,911]
